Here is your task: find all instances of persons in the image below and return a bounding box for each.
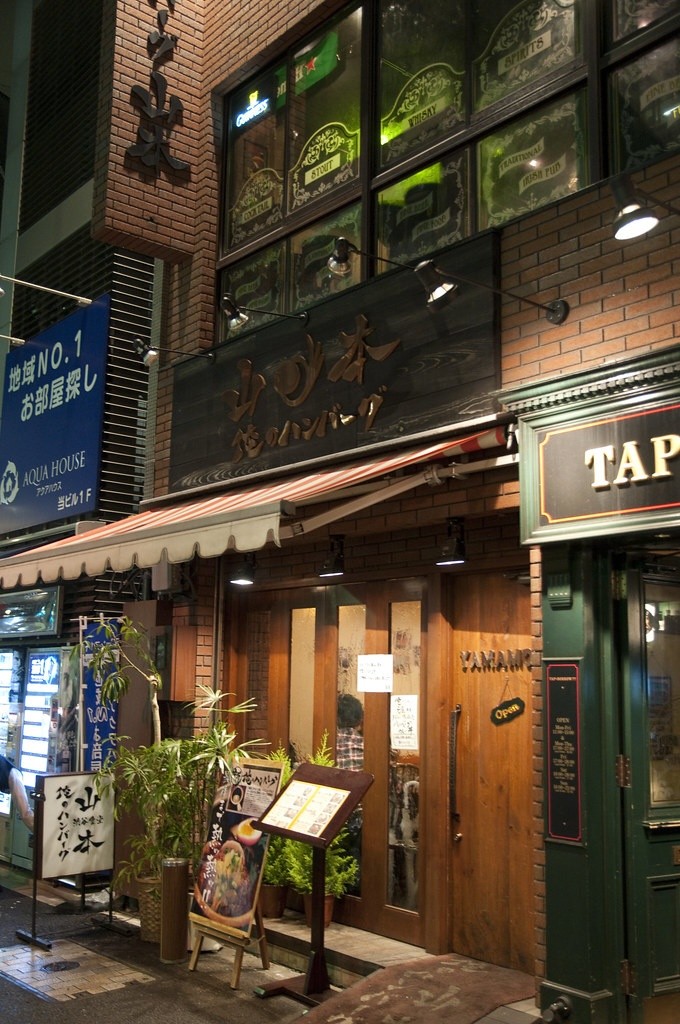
[0,755,34,833]
[334,694,399,868]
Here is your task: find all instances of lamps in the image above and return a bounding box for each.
[319,537,344,577]
[414,262,570,325]
[219,297,309,330]
[327,237,415,275]
[436,521,467,566]
[134,339,216,366]
[609,173,680,241]
[229,554,256,585]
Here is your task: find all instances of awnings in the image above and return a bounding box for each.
[0,425,508,589]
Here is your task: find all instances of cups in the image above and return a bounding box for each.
[231,786,243,811]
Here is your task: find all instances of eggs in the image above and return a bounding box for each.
[237,817,262,845]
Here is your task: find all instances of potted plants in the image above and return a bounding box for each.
[67,615,359,943]
